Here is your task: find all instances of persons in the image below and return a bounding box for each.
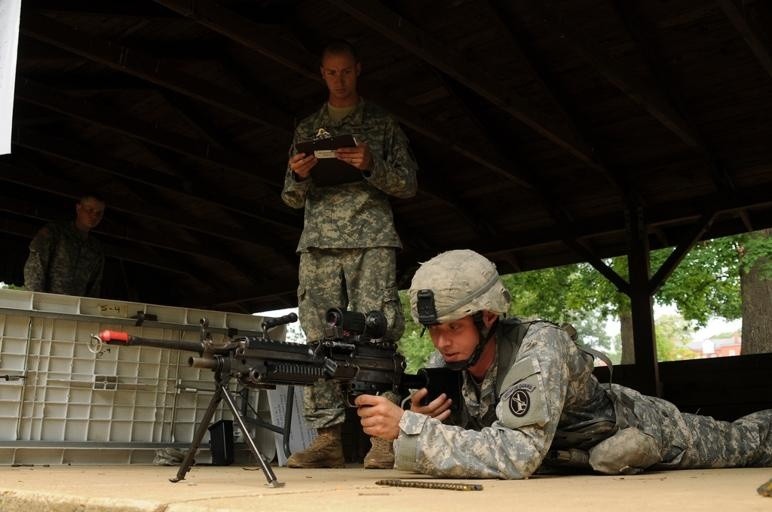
[16,190,109,297]
[272,41,423,475]
[352,249,772,478]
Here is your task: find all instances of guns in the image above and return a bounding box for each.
[88,306,464,487]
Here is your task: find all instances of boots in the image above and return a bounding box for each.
[287,424,345,469]
[364,436,394,469]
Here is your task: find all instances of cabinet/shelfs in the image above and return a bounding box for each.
[1,290,288,466]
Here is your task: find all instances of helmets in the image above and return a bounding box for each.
[407,249,512,325]
